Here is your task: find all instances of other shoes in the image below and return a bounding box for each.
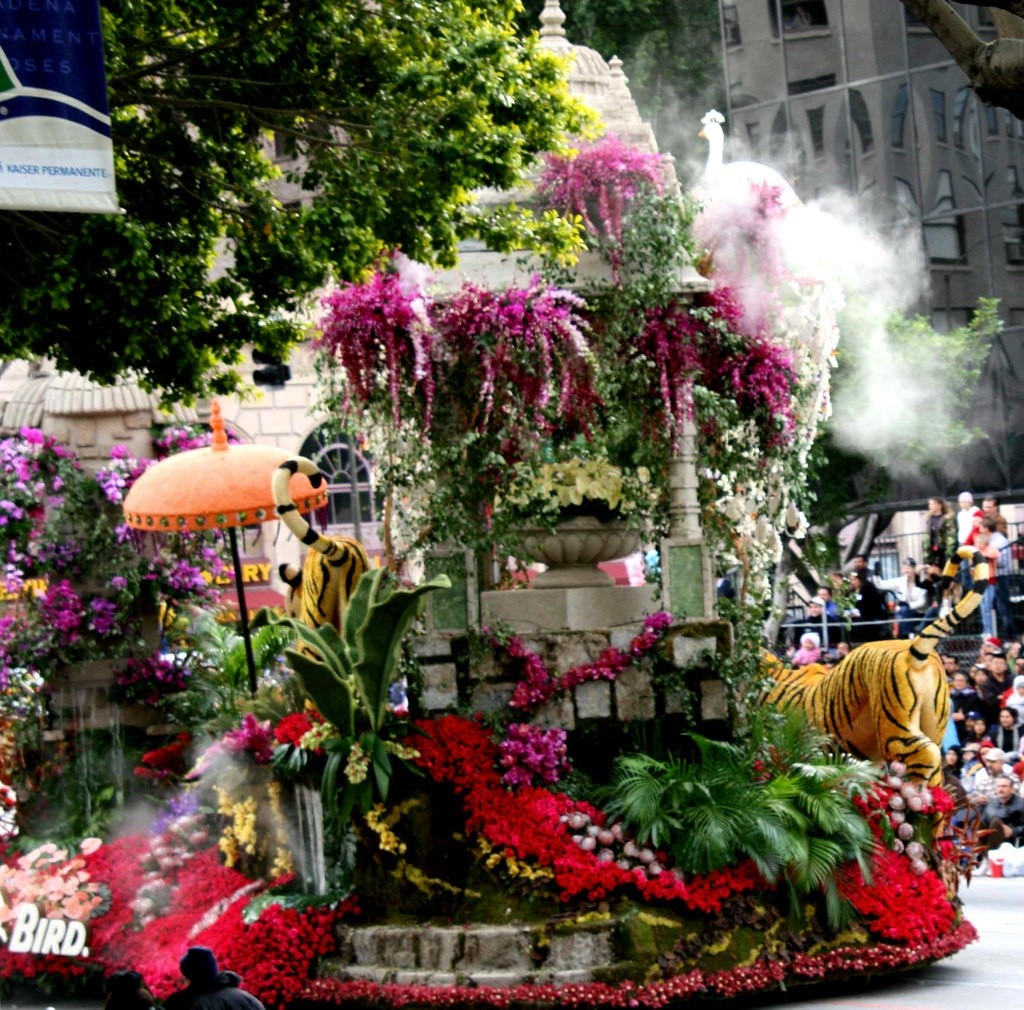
[971,858,989,876]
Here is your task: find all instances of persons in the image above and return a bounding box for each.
[786,490,1012,671]
[938,640,1024,877]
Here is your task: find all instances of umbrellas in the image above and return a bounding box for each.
[123,400,330,700]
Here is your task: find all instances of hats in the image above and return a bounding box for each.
[800,631,820,648]
[965,711,980,720]
[960,742,979,752]
[986,638,1003,651]
[810,596,826,607]
[980,741,995,749]
[982,748,1006,761]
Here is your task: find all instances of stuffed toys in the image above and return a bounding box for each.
[272,456,371,710]
[735,545,989,847]
